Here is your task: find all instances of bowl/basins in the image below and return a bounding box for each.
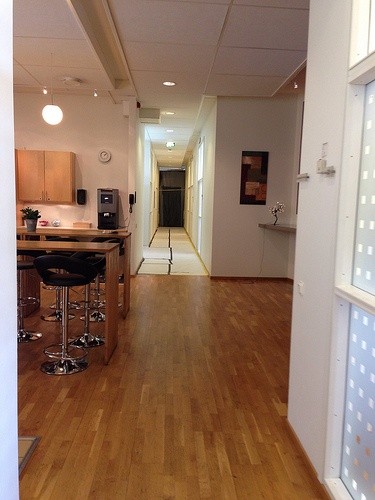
[38,220,47,226]
[51,220,61,227]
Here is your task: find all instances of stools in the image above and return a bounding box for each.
[16,236,124,376]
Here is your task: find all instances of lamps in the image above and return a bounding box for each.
[42,81,64,126]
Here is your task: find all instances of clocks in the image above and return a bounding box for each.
[98,149,111,163]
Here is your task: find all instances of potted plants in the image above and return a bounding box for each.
[20,206,41,232]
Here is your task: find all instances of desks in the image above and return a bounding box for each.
[16,240,120,365]
[16,226,132,320]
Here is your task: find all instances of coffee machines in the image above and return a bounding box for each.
[96,187,119,230]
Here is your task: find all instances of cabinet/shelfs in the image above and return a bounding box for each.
[16,149,75,205]
